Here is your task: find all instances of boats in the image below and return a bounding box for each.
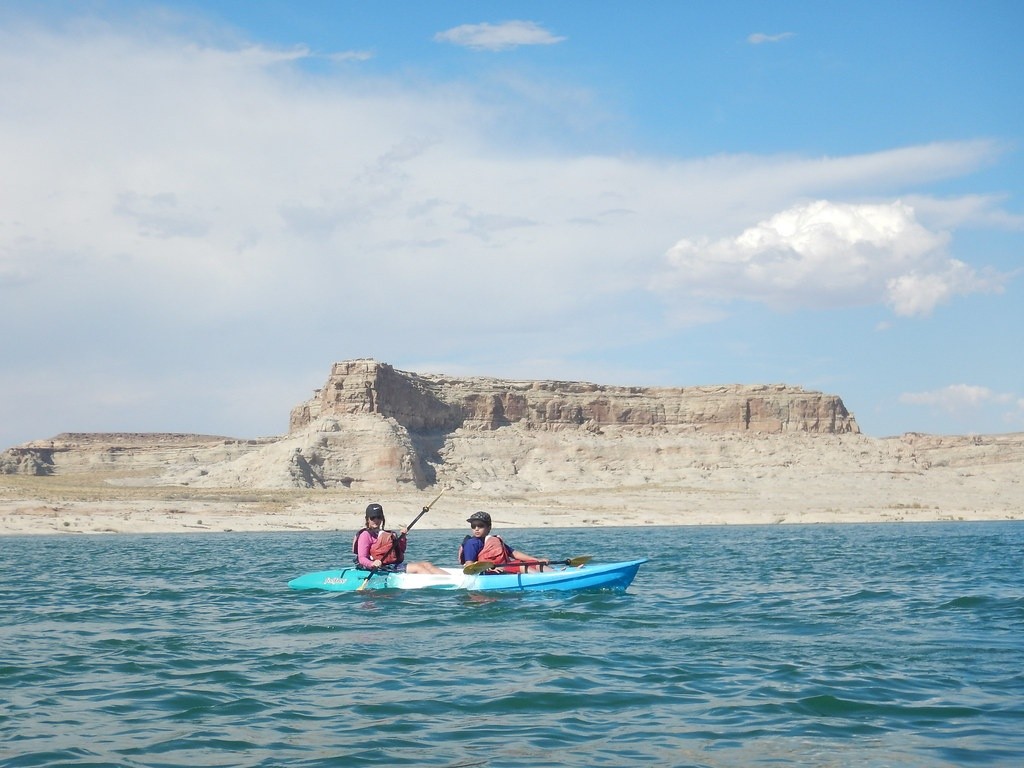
[287,556,646,596]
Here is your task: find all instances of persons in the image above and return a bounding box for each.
[356,503,452,575]
[463,511,584,575]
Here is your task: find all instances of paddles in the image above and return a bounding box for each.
[355,488,446,590]
[463,553,594,576]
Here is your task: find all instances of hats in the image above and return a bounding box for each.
[365,504,383,518]
[466,511,491,526]
